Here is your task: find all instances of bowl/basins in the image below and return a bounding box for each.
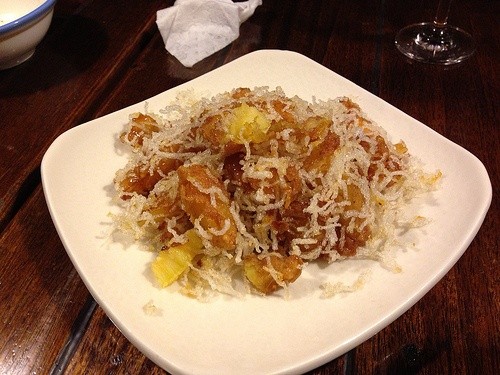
[0,0,54,71]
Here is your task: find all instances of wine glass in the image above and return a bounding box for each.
[395,0,477,64]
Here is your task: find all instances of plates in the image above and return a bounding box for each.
[41,50,492,374]
